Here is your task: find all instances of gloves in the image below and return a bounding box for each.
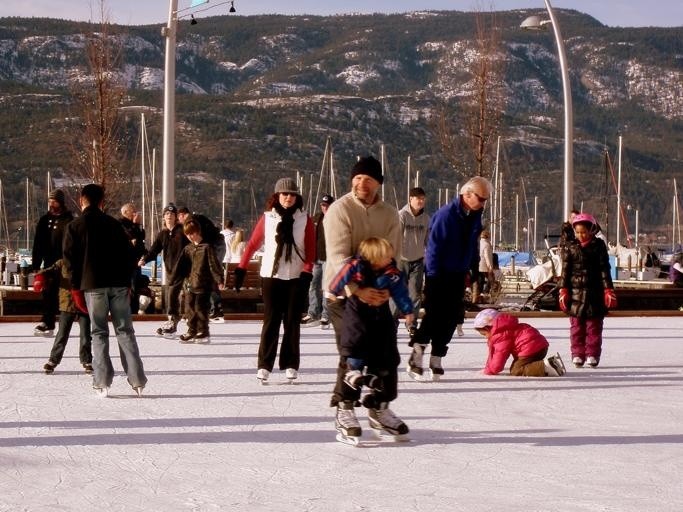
[559,288,571,311]
[300,272,312,297]
[235,268,246,292]
[71,290,88,313]
[604,288,618,309]
[33,275,45,292]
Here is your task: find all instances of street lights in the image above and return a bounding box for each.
[517,0,574,224]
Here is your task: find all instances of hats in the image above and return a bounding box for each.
[163,202,177,217]
[320,194,333,205]
[674,244,683,254]
[351,155,383,185]
[49,189,64,201]
[275,178,299,193]
[410,188,425,196]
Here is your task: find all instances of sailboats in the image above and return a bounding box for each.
[135,112,164,280]
[1,171,56,264]
[656,177,682,269]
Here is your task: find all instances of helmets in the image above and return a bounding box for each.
[572,213,596,228]
[474,309,498,329]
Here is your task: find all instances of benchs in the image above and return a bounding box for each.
[219,261,260,300]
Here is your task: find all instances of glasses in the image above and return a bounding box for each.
[475,193,486,202]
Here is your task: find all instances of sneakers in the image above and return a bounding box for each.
[300,315,310,321]
[335,371,408,436]
[256,369,269,380]
[44,362,57,369]
[457,324,463,335]
[573,357,583,364]
[544,357,561,376]
[286,368,297,379]
[83,364,93,370]
[586,356,597,365]
[408,327,444,375]
[36,322,55,330]
[157,309,223,340]
[320,318,329,323]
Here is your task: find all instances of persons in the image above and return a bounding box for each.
[139,201,247,343]
[33,190,148,398]
[405,176,488,373]
[330,236,416,392]
[475,208,682,383]
[322,157,409,437]
[396,186,434,333]
[233,178,318,380]
[301,193,330,323]
